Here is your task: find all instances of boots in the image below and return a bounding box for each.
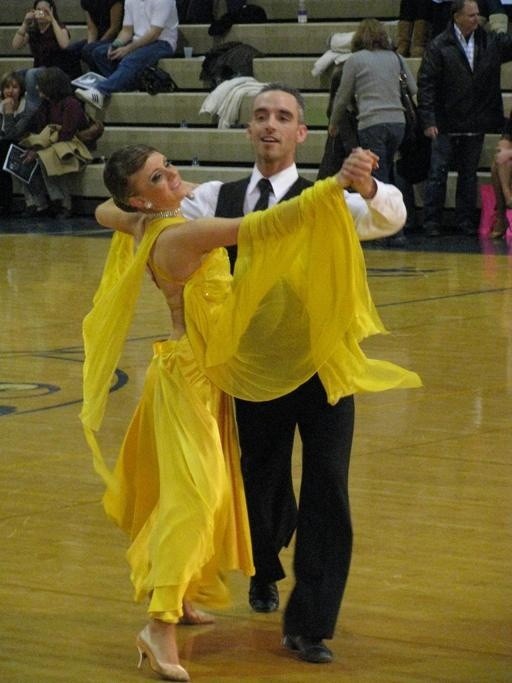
[409,19,430,57]
[395,21,414,56]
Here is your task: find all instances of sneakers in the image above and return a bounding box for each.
[75,87,104,111]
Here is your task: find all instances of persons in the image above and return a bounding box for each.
[175,83,409,664]
[95,146,380,678]
[316,1,511,241]
[1,2,261,227]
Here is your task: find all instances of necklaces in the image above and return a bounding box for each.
[142,208,184,220]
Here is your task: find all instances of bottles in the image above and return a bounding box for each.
[297,0,308,24]
[192,154,200,166]
[98,155,107,164]
[180,119,189,127]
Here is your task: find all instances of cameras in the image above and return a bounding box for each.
[34,10,45,18]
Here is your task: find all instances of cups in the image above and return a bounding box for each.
[184,46,193,58]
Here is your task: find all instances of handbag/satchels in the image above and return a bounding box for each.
[399,81,419,138]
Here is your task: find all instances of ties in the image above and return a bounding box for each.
[252,178,272,211]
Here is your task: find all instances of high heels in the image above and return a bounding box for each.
[136,624,191,681]
[184,608,214,623]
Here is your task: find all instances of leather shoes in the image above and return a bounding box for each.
[249,577,279,612]
[487,218,509,239]
[424,222,441,236]
[281,634,333,663]
